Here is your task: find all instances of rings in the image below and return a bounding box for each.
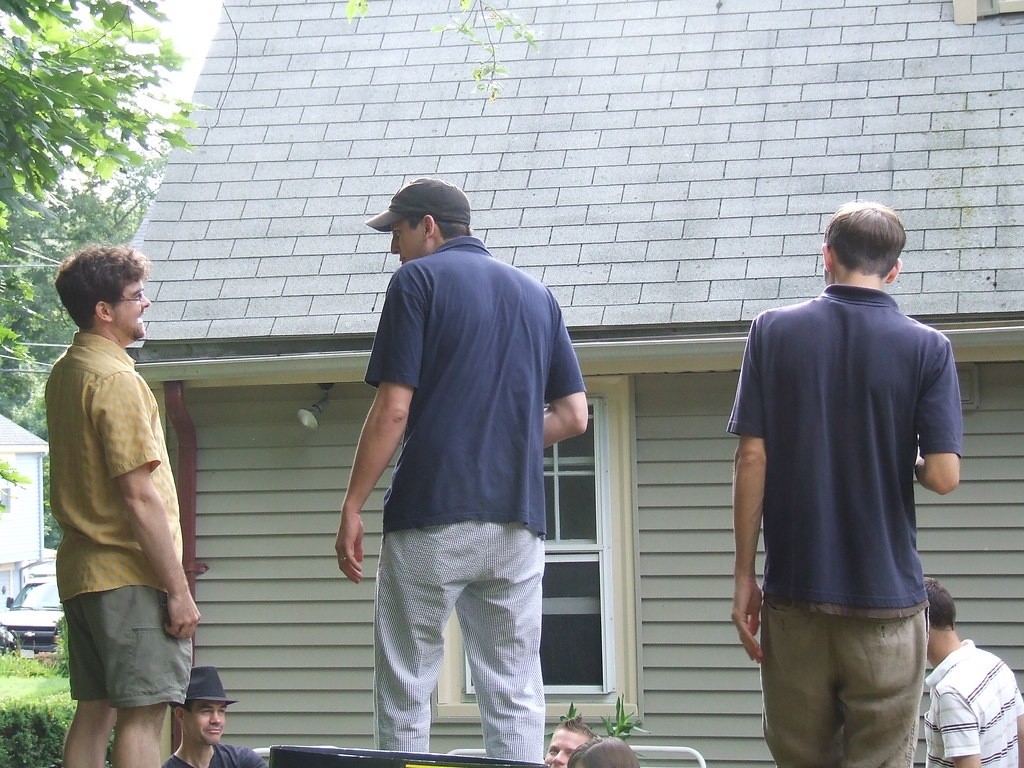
[341,557,346,561]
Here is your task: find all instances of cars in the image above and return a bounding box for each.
[0,582,65,651]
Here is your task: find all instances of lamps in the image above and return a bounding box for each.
[297,383,335,428]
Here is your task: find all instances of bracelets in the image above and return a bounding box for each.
[169,586,189,602]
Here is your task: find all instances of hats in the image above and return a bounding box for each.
[364,178,471,232]
[166,666,238,705]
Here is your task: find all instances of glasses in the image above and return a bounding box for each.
[92,289,146,313]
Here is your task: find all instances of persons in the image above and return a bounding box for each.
[44,240,201,768]
[727,204,963,768]
[567,737,640,768]
[544,715,595,768]
[161,666,268,768]
[335,179,589,765]
[923,578,1024,768]
